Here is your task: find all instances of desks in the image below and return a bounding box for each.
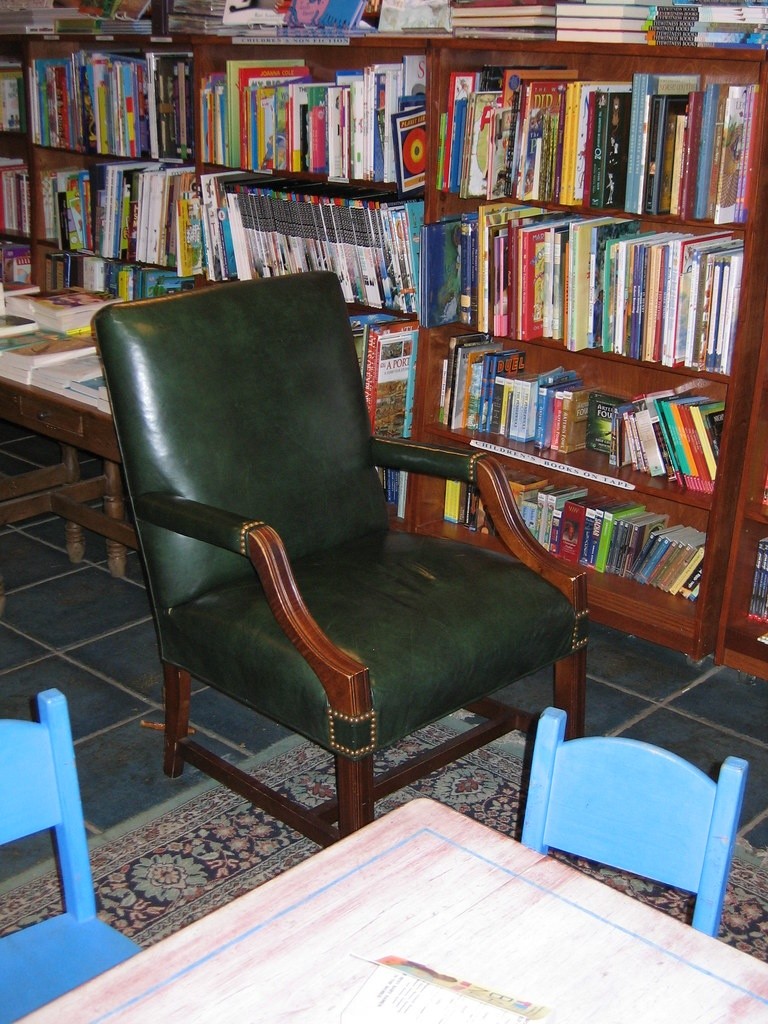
[0,378,142,579]
[10,797,768,1024]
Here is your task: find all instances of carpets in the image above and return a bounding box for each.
[0,710,768,965]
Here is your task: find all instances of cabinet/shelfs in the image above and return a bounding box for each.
[0,1,768,682]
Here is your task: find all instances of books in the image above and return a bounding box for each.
[0,0,768,627]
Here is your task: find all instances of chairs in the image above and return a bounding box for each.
[91,272,589,848]
[0,688,142,1024]
[521,707,748,938]
